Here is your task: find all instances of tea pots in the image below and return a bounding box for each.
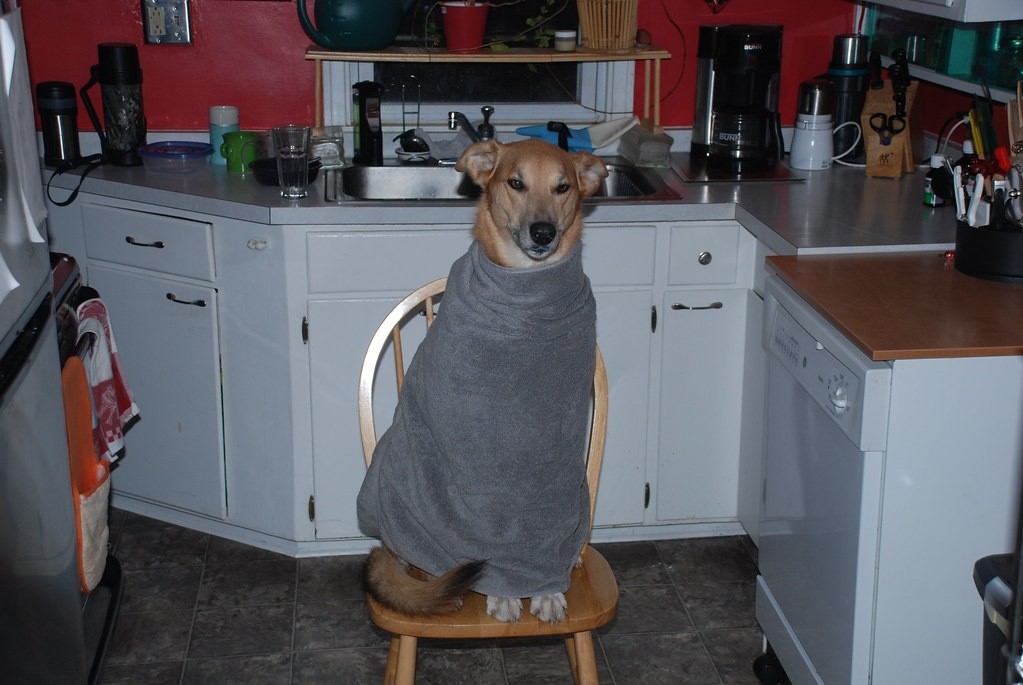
[297,0,413,51]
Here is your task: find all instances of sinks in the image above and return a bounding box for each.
[580,162,682,202]
[340,163,484,201]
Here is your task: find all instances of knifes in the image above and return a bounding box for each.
[870,47,910,117]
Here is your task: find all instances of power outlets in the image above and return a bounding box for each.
[140,0,192,45]
[965,96,994,132]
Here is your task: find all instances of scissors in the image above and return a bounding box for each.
[869,112,906,144]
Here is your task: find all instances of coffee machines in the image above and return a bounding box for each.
[690,25,784,179]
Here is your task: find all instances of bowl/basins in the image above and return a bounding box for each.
[396,148,430,161]
[136,141,216,176]
[248,160,322,187]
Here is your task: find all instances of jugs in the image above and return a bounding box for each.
[81,44,147,167]
[823,34,869,159]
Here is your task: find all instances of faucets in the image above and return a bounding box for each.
[446,103,496,143]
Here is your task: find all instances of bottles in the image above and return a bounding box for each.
[555,31,576,52]
[999,36,1023,91]
[35,82,81,170]
[953,140,982,200]
[924,155,946,207]
[272,125,309,157]
[352,81,385,166]
[210,107,239,165]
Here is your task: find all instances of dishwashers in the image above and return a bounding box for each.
[756,276,1023,685]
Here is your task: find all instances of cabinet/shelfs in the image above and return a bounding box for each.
[288,223,660,556]
[43,187,296,563]
[651,220,757,540]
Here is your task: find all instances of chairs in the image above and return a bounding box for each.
[359,277,619,685]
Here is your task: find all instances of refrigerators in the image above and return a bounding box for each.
[0,6,84,685]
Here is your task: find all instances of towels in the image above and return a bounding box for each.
[352,235,598,599]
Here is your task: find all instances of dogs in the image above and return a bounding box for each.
[363,138,611,624]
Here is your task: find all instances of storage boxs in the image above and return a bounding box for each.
[134,141,217,177]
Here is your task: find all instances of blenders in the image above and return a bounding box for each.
[789,82,866,170]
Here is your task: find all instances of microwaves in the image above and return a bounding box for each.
[863,0,1023,23]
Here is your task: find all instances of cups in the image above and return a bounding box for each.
[221,133,275,174]
[908,36,925,66]
[277,147,309,199]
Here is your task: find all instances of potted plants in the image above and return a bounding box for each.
[411,0,569,55]
